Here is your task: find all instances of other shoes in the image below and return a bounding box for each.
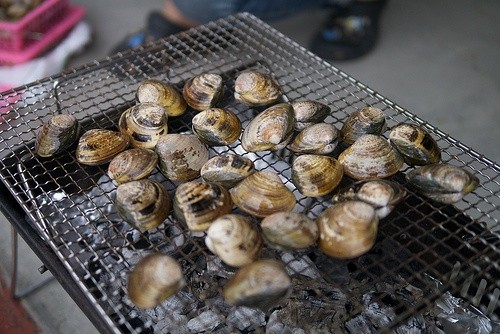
[103,9,232,81]
[308,11,379,63]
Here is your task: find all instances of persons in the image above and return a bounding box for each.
[102,0,388,79]
